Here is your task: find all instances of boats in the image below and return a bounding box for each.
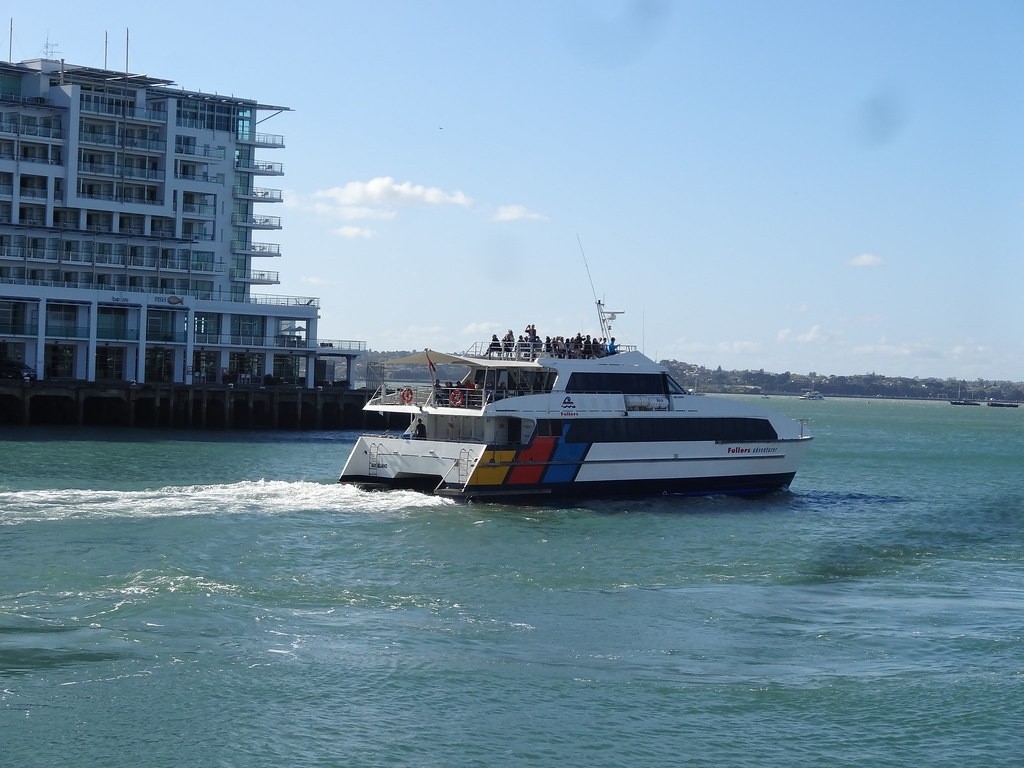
[950,399,980,405]
[988,401,1020,407]
[334,342,816,504]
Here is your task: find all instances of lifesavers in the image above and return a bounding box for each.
[401,388,414,403]
[449,390,463,407]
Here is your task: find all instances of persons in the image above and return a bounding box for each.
[434,377,477,403]
[415,418,427,440]
[491,381,524,401]
[483,324,620,360]
[447,423,456,440]
